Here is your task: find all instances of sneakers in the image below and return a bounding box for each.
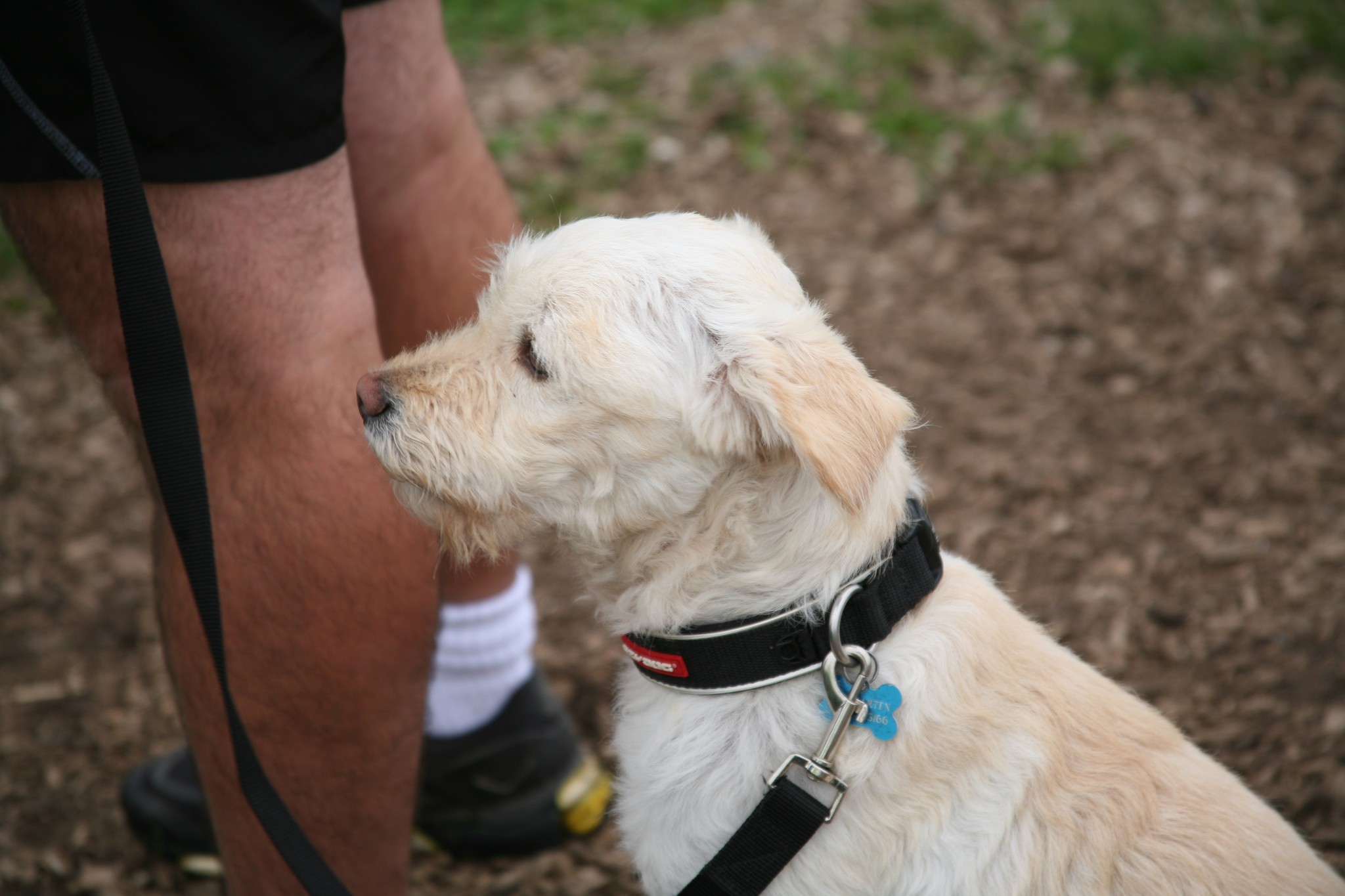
[117,664,613,879]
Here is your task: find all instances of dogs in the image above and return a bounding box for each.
[357,209,1344,896]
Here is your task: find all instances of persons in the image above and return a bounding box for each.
[0,0,608,896]
